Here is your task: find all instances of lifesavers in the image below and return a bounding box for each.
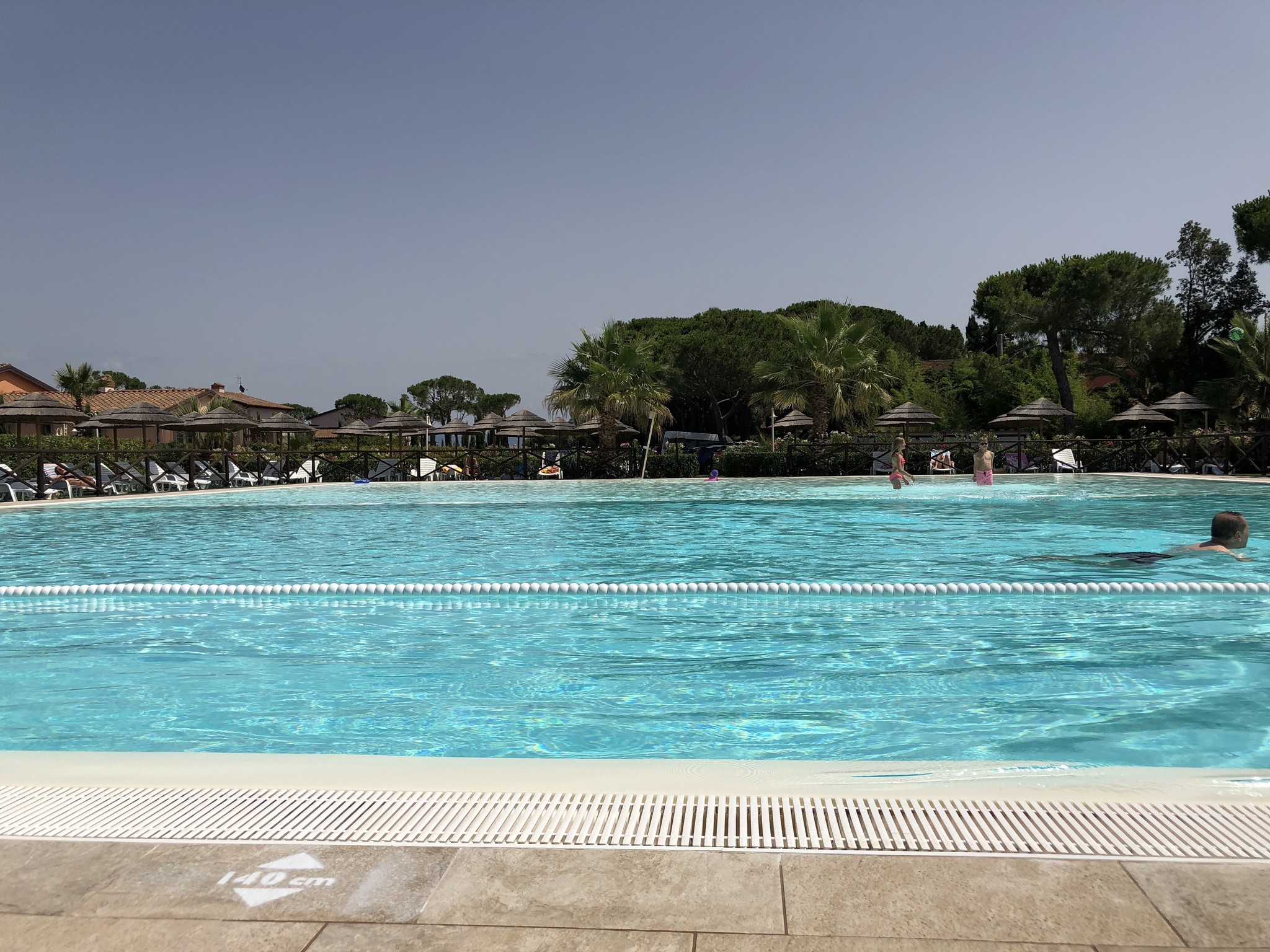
[354,478,369,484]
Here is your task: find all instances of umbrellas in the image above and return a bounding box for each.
[766,409,813,449]
[363,410,640,480]
[872,392,1217,473]
[331,418,384,460]
[0,391,90,494]
[73,402,318,496]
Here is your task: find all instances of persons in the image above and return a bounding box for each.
[1023,511,1253,567]
[53,466,97,488]
[972,436,995,486]
[889,437,916,490]
[704,469,719,481]
[807,429,814,441]
[500,468,511,480]
[1204,456,1226,466]
[1155,451,1172,465]
[929,454,955,468]
[462,451,484,480]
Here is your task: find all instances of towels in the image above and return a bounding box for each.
[543,451,559,467]
[443,467,463,475]
[541,467,559,473]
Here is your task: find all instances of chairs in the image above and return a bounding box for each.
[407,458,437,481]
[440,464,470,481]
[1052,449,1084,473]
[870,452,892,475]
[0,460,324,502]
[927,449,955,474]
[368,459,400,481]
[537,452,563,479]
[1005,453,1039,473]
[1141,451,1233,475]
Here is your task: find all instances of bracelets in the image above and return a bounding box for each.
[909,474,911,477]
[974,476,977,477]
[911,476,913,479]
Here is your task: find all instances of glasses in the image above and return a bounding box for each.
[980,443,988,445]
